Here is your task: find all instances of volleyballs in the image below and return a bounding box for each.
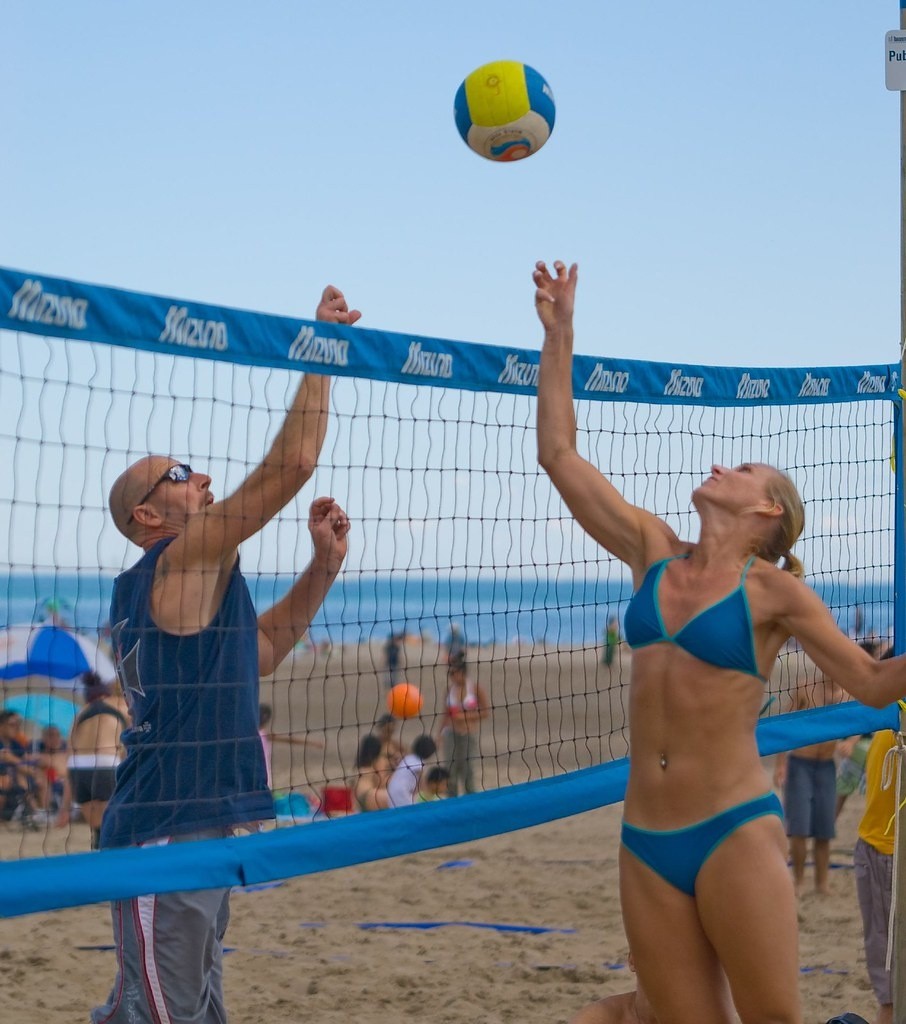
[453,59,557,163]
[387,683,423,717]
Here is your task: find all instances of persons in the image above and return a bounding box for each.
[593,614,906,1024]
[90,285,363,1024]
[533,258,906,1024]
[66,669,132,852]
[0,710,80,829]
[257,620,492,816]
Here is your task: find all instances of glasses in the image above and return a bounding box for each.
[126,464,193,525]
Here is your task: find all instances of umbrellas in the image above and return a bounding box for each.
[0,608,120,705]
